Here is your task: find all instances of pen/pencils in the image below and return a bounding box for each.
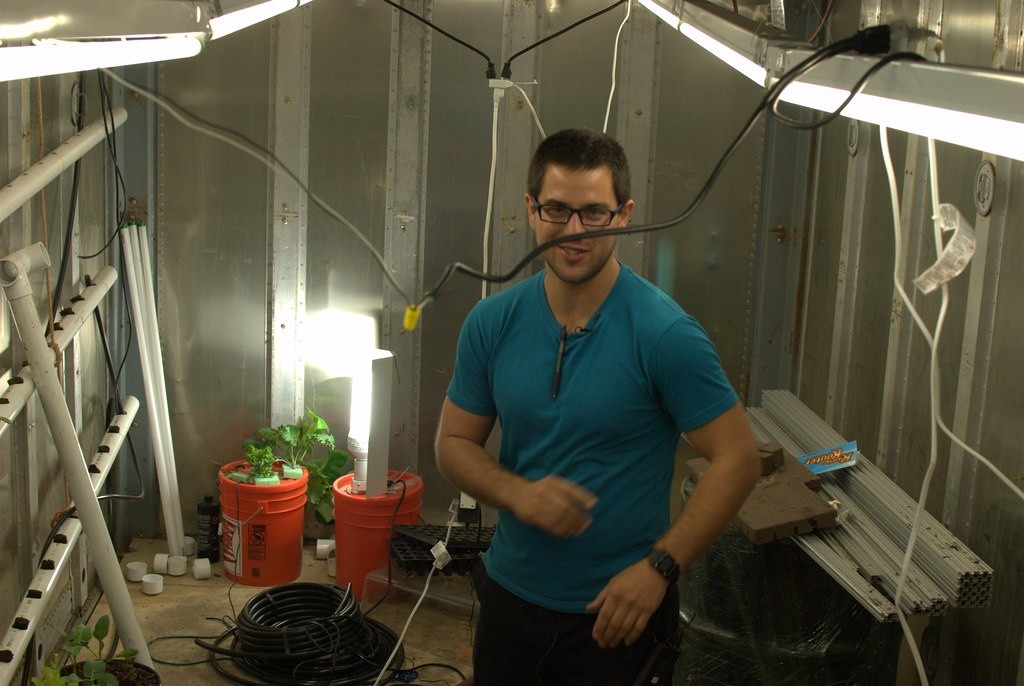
[549,324,568,398]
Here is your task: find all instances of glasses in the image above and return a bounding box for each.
[530,195,623,227]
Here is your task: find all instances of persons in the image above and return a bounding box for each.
[435,129,762,686]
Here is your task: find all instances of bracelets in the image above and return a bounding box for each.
[645,548,681,596]
